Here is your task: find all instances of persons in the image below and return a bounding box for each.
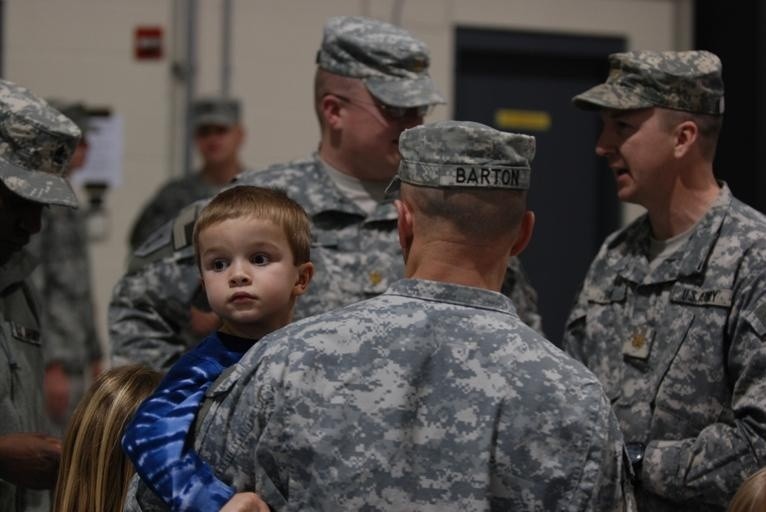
[124,122,636,512]
[108,17,544,375]
[0,76,81,512]
[119,185,314,512]
[31,99,102,426]
[131,97,247,248]
[728,466,766,512]
[562,50,766,512]
[52,362,162,512]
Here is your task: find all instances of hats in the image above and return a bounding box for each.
[316,15,447,108]
[195,100,239,130]
[0,80,81,209]
[573,50,717,116]
[383,120,536,196]
